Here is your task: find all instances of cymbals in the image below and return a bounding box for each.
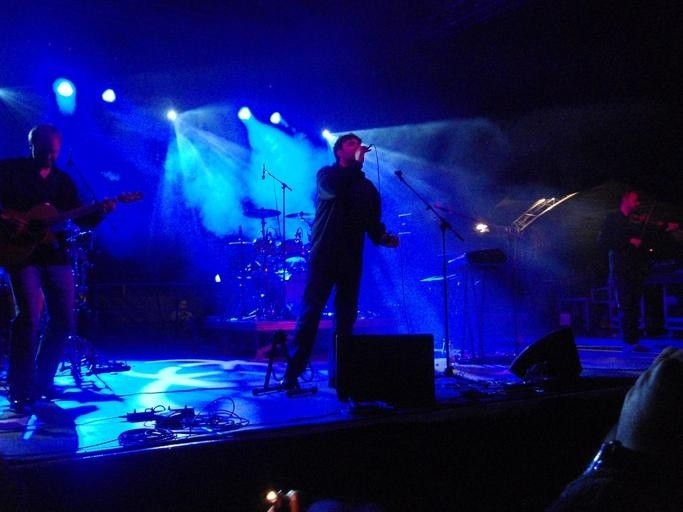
[243,209,281,218]
[285,212,310,218]
[227,241,252,247]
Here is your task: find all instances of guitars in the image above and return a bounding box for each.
[0,191,144,265]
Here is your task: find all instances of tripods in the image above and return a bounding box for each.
[434,222,492,388]
[56,243,131,387]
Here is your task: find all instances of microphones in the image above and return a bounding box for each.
[66,147,74,168]
[393,169,402,176]
[67,230,92,242]
[261,163,266,180]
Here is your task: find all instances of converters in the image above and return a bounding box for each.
[153,409,183,426]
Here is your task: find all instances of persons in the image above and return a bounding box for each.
[538,340,681,511]
[171,297,195,320]
[599,183,680,343]
[272,133,399,383]
[2,123,114,409]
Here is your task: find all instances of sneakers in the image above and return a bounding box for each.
[280,377,297,389]
[10,383,66,415]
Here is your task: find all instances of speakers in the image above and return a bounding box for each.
[336,333,436,405]
[508,326,582,383]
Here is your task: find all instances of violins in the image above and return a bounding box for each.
[628,209,679,232]
